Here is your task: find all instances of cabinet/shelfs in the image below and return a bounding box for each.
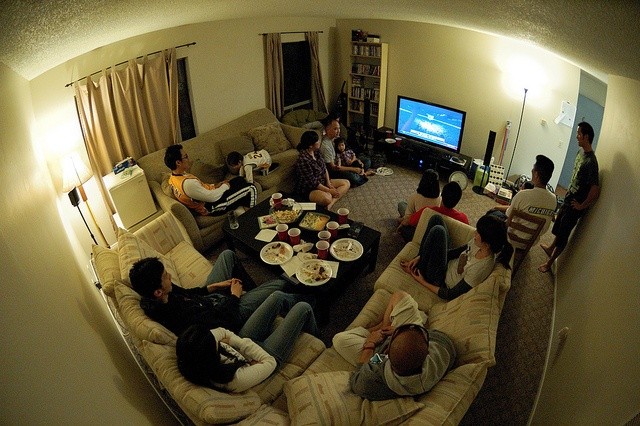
[100,165,158,230]
[373,126,473,184]
[346,40,389,130]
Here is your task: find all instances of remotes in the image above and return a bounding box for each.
[352,222,365,238]
[346,221,359,237]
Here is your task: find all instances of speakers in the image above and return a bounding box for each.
[483,129,496,167]
[363,98,371,136]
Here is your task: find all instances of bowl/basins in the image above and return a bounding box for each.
[269,199,303,224]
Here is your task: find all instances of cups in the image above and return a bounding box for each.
[227,210,240,230]
[272,193,282,204]
[395,137,402,149]
[325,221,339,239]
[386,130,393,138]
[276,224,289,242]
[288,228,302,246]
[318,231,332,244]
[316,241,330,260]
[337,208,349,225]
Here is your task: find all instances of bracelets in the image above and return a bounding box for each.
[361,344,375,351]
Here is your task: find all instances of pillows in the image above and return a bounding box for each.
[161,171,187,200]
[90,241,121,298]
[282,370,425,426]
[217,135,255,155]
[115,227,182,288]
[234,401,289,425]
[139,339,262,425]
[250,120,292,155]
[111,279,178,347]
[493,252,511,296]
[427,275,498,369]
[394,358,490,425]
[190,158,225,184]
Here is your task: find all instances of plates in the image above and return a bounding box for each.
[385,138,396,144]
[377,166,394,176]
[258,215,277,229]
[260,241,294,265]
[296,259,333,286]
[330,238,364,262]
[299,212,330,232]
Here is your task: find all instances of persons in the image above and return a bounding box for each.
[332,291,457,401]
[538,121,600,273]
[175,290,318,393]
[164,144,258,217]
[334,137,364,175]
[227,149,279,184]
[129,249,257,337]
[401,181,469,226]
[486,154,558,250]
[293,131,351,210]
[399,214,515,301]
[320,119,376,188]
[398,169,442,227]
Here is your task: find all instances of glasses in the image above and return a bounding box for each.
[389,323,430,350]
[179,154,188,160]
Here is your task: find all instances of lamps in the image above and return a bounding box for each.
[44,149,110,251]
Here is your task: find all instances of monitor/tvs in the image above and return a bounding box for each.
[395,94,466,153]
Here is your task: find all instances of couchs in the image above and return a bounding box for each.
[282,109,348,149]
[90,210,326,406]
[236,207,516,426]
[136,107,322,255]
